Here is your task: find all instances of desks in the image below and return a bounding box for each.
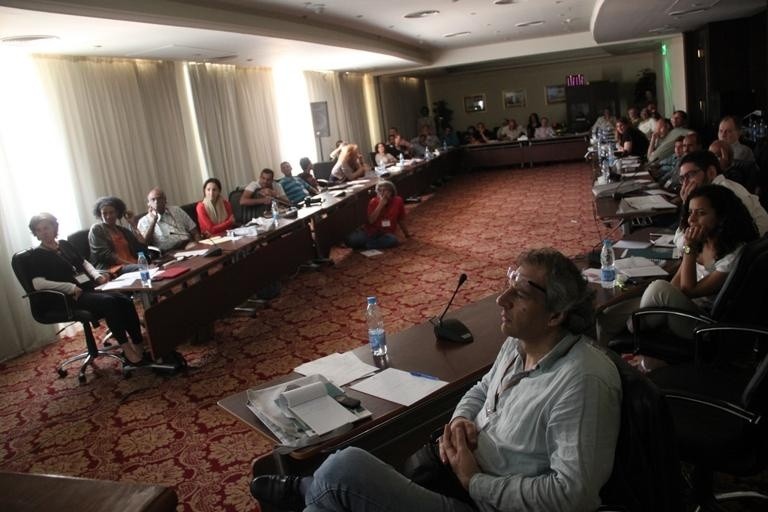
[590,133,681,230]
[97,149,449,371]
[216,230,680,476]
[448,133,595,174]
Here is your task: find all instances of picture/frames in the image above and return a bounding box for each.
[543,85,566,104]
[463,94,487,113]
[501,88,527,110]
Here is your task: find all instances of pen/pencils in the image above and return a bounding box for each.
[411,371,439,382]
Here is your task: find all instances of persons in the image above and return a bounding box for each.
[137,188,201,255]
[587,102,760,236]
[329,140,348,160]
[422,125,441,147]
[250,245,623,512]
[296,157,319,196]
[610,184,760,354]
[240,168,290,208]
[374,143,398,167]
[28,212,144,367]
[410,135,426,156]
[442,108,581,141]
[385,135,404,160]
[385,127,410,147]
[196,177,236,238]
[87,197,151,273]
[327,144,367,187]
[278,161,319,205]
[343,180,413,250]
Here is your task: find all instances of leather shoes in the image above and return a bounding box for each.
[122,348,148,367]
[249,472,303,504]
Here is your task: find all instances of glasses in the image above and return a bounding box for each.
[679,168,700,185]
[506,265,549,296]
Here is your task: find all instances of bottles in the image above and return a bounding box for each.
[443,140,447,150]
[598,137,602,156]
[270,198,280,227]
[137,252,152,289]
[399,152,405,168]
[365,296,387,356]
[424,146,430,162]
[600,238,616,289]
[378,160,385,171]
[606,142,614,165]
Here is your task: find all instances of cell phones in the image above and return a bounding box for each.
[334,394,360,409]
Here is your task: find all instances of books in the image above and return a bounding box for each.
[612,256,670,277]
[624,194,677,210]
[154,254,177,267]
[153,267,191,278]
[274,381,372,438]
[628,247,673,260]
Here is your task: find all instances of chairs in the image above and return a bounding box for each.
[67,230,90,260]
[753,136,768,171]
[12,240,133,382]
[605,232,768,512]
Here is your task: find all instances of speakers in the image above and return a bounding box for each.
[309,101,331,137]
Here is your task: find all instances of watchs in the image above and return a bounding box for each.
[682,245,697,254]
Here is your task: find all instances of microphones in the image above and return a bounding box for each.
[588,215,628,269]
[429,273,473,345]
[612,157,659,201]
[169,232,222,258]
